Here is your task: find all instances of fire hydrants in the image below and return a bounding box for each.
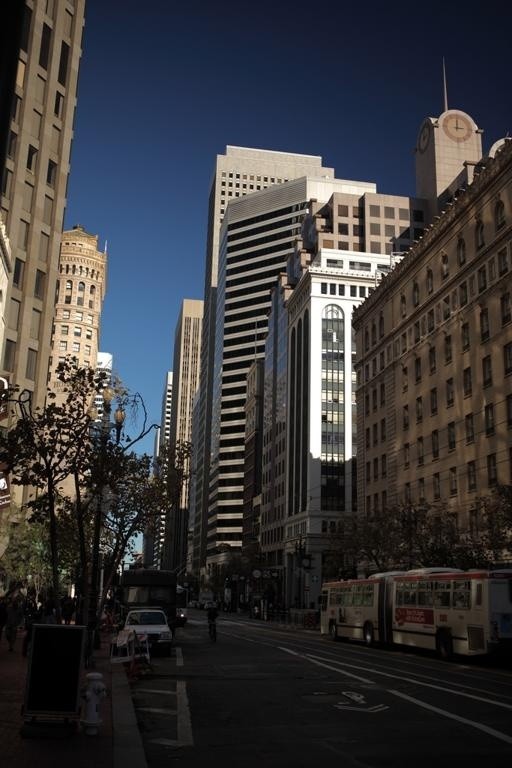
[80,671,110,735]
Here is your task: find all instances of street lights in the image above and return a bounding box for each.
[84,387,126,666]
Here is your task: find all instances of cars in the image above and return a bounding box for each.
[123,608,173,655]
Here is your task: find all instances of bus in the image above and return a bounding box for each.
[317,566,511,663]
[173,585,189,626]
[117,567,175,629]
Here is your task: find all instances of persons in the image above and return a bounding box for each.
[1,582,78,658]
[206,602,219,642]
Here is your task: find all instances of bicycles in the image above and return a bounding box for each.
[208,621,217,641]
